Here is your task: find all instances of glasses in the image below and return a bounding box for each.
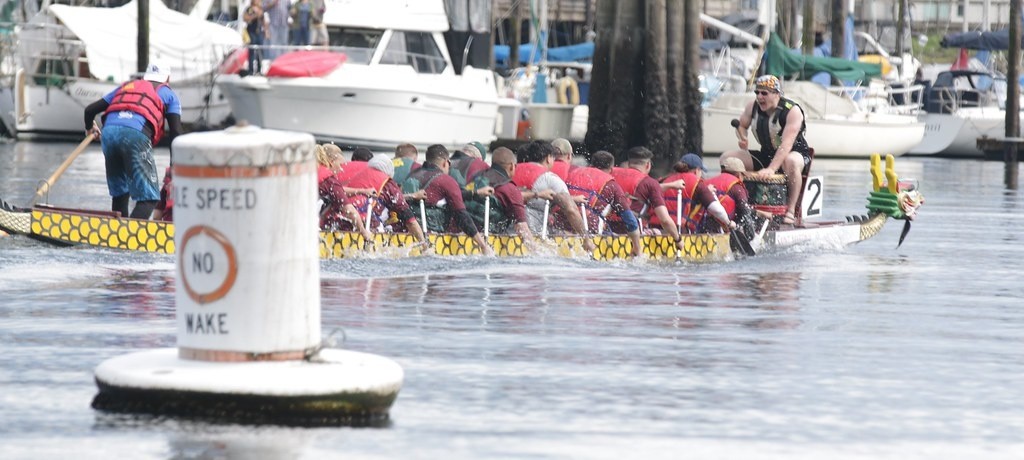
[754,90,772,96]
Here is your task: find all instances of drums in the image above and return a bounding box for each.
[743,171,788,220]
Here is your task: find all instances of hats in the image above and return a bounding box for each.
[550,138,576,159]
[721,157,748,176]
[680,153,708,173]
[143,59,170,83]
[367,154,394,179]
[468,142,486,161]
[757,74,785,97]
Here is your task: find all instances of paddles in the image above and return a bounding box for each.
[360,181,775,261]
[24,132,96,211]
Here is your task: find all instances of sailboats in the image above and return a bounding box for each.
[700,0,1024,160]
[497,1,594,147]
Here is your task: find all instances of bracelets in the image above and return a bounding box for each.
[673,234,681,243]
[728,221,730,226]
[535,193,538,198]
[474,189,476,194]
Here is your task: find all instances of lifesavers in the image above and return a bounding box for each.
[555,76,581,106]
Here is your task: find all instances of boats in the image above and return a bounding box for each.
[0,151,925,268]
[0,1,248,143]
[209,0,501,158]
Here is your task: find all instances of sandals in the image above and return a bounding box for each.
[783,210,795,224]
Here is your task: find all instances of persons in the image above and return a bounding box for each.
[719,75,811,224]
[153,167,173,221]
[611,147,685,251]
[317,143,378,242]
[402,144,495,254]
[555,151,641,255]
[84,58,186,219]
[344,152,428,249]
[510,139,596,252]
[243,0,329,76]
[346,138,630,187]
[467,146,556,254]
[704,156,758,249]
[648,153,736,232]
